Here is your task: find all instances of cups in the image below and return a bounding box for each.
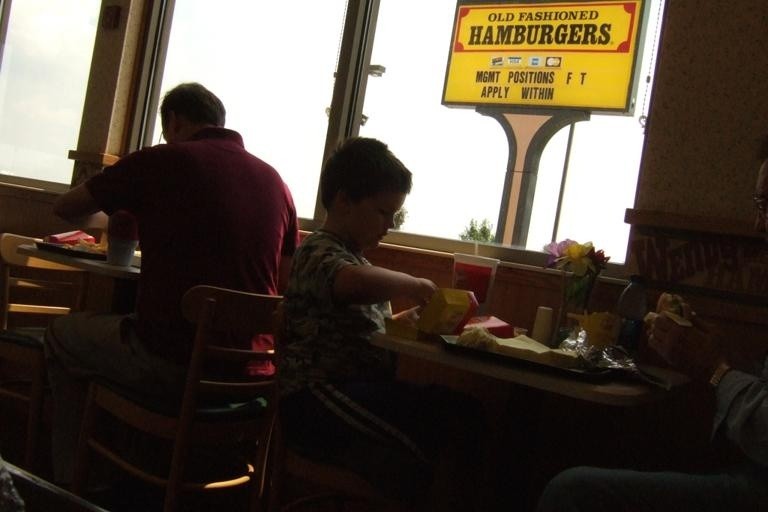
[531,306,555,347]
[452,252,499,319]
[108,229,139,268]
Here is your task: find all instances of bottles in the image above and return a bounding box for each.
[616,274,648,355]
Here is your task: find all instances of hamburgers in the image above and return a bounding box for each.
[655,293,694,323]
[397,310,417,326]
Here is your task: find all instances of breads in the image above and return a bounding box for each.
[459,326,554,365]
[554,344,600,369]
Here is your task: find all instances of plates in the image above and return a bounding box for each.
[34,239,112,261]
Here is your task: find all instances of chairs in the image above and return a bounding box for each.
[26,287,287,512]
[0,232,90,474]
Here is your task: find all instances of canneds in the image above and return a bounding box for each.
[588,311,621,347]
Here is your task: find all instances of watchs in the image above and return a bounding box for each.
[707,360,731,396]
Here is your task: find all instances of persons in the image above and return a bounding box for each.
[535,138,767,511]
[45,80,300,511]
[272,137,445,510]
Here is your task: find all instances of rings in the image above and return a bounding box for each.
[671,321,677,329]
[659,330,667,342]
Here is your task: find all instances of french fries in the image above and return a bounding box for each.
[63,239,108,252]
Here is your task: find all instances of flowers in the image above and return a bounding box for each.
[544,239,612,315]
